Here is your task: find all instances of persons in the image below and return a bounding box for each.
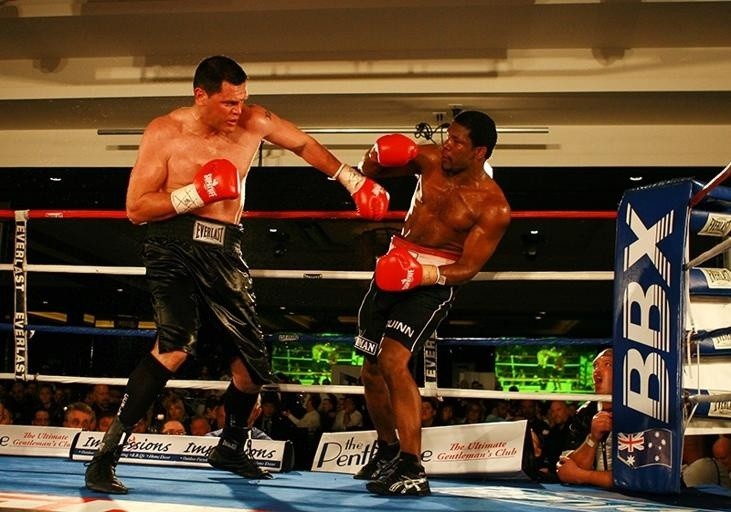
[63,384,272,441]
[256,391,364,435]
[684,434,731,490]
[345,108,512,497]
[421,380,578,484]
[82,56,395,494]
[555,349,613,488]
[2,380,68,426]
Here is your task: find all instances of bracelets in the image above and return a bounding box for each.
[586,435,595,447]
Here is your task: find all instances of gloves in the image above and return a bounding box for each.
[333,163,390,223]
[170,158,240,216]
[358,133,419,177]
[374,248,441,292]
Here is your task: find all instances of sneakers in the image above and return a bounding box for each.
[354,438,401,480]
[366,451,430,498]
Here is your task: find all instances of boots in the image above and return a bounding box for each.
[208,425,273,480]
[84,415,133,495]
